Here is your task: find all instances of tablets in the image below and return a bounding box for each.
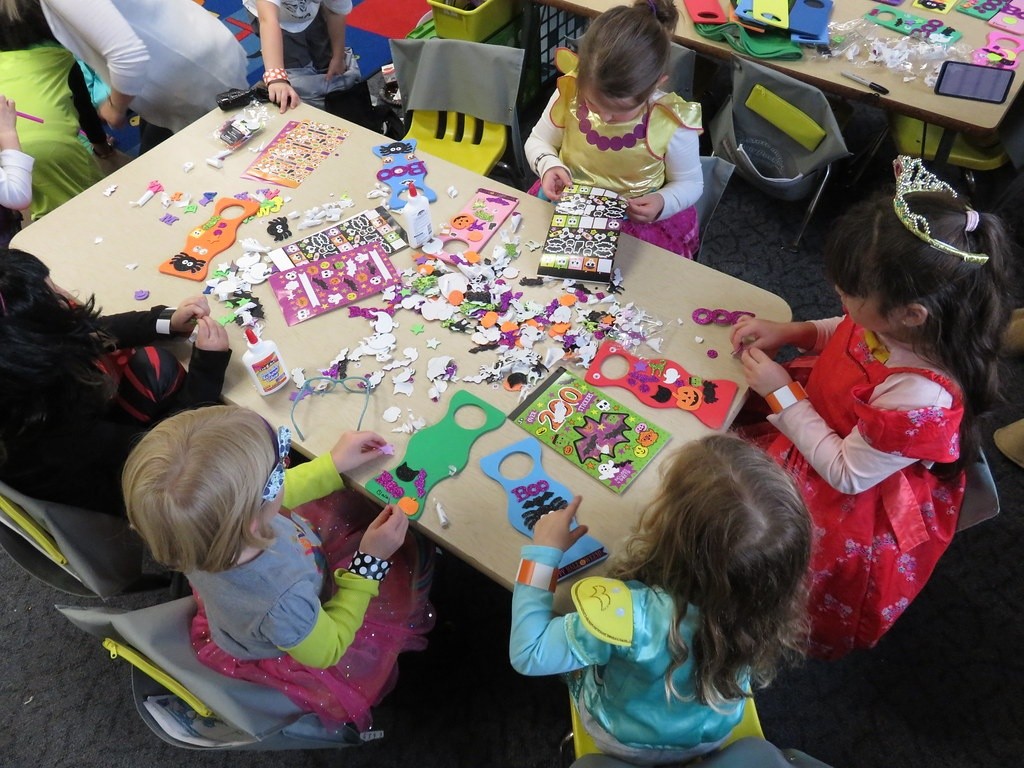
[935,61,1016,105]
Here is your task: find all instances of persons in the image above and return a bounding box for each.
[121,404,437,737]
[509,434,819,768]
[0,0,249,240]
[242,0,352,114]
[524,0,705,262]
[0,249,233,520]
[730,155,1012,663]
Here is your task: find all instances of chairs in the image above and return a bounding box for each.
[855,109,1010,197]
[385,39,847,269]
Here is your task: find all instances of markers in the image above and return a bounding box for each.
[840,70,889,95]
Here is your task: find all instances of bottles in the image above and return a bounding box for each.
[242,329,290,396]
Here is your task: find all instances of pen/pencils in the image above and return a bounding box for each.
[16,111,44,123]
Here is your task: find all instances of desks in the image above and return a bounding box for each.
[539,0,1024,178]
[12,102,792,617]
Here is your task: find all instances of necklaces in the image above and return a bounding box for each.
[576,94,649,151]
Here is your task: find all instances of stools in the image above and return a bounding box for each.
[568,681,765,761]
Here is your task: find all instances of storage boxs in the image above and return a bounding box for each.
[427,0,514,42]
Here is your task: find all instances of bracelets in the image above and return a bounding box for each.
[348,551,394,582]
[156,307,178,335]
[263,68,291,87]
[534,152,557,176]
[765,381,809,415]
[514,558,559,593]
